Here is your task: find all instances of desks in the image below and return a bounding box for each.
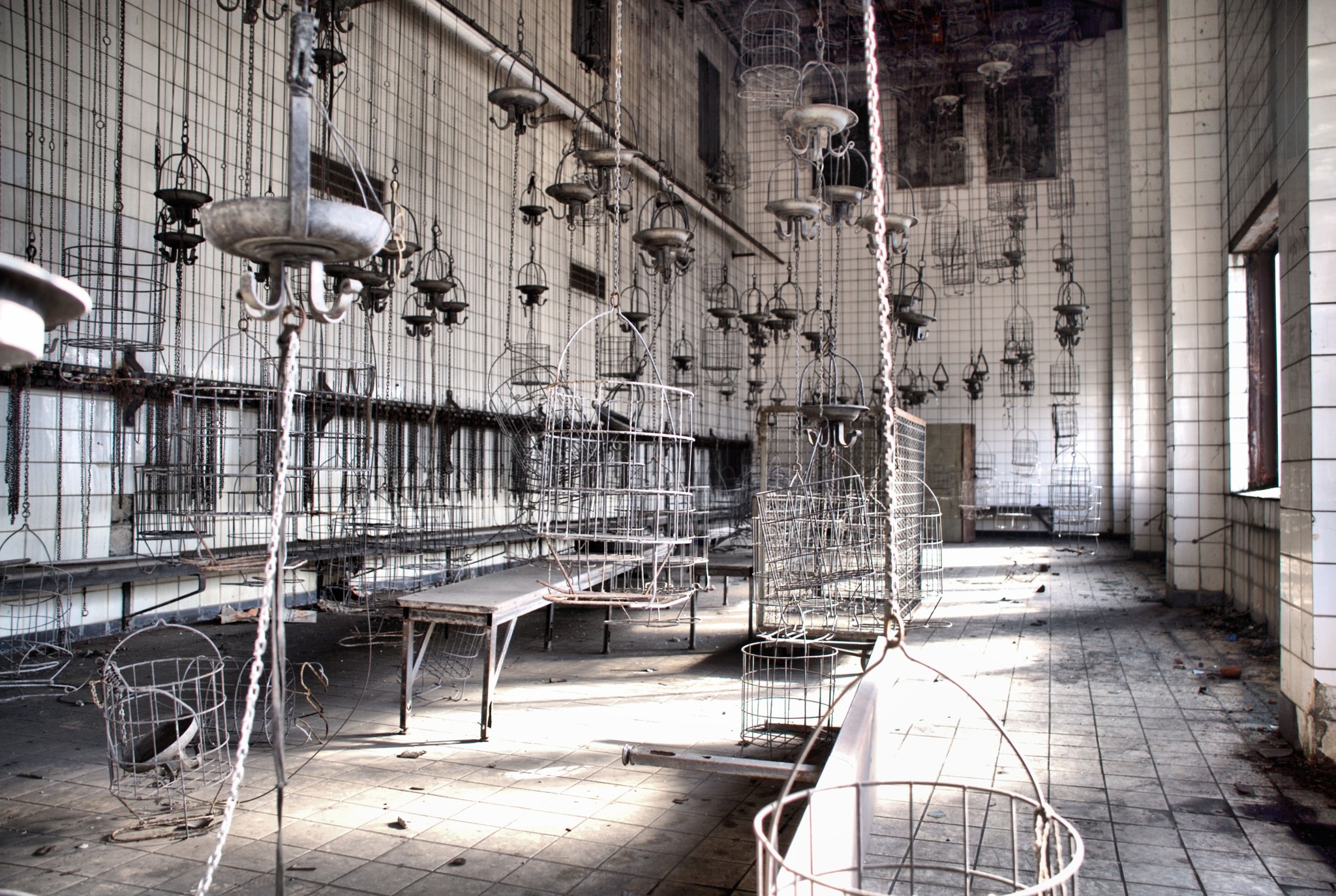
[395,553,644,743]
[687,552,785,649]
